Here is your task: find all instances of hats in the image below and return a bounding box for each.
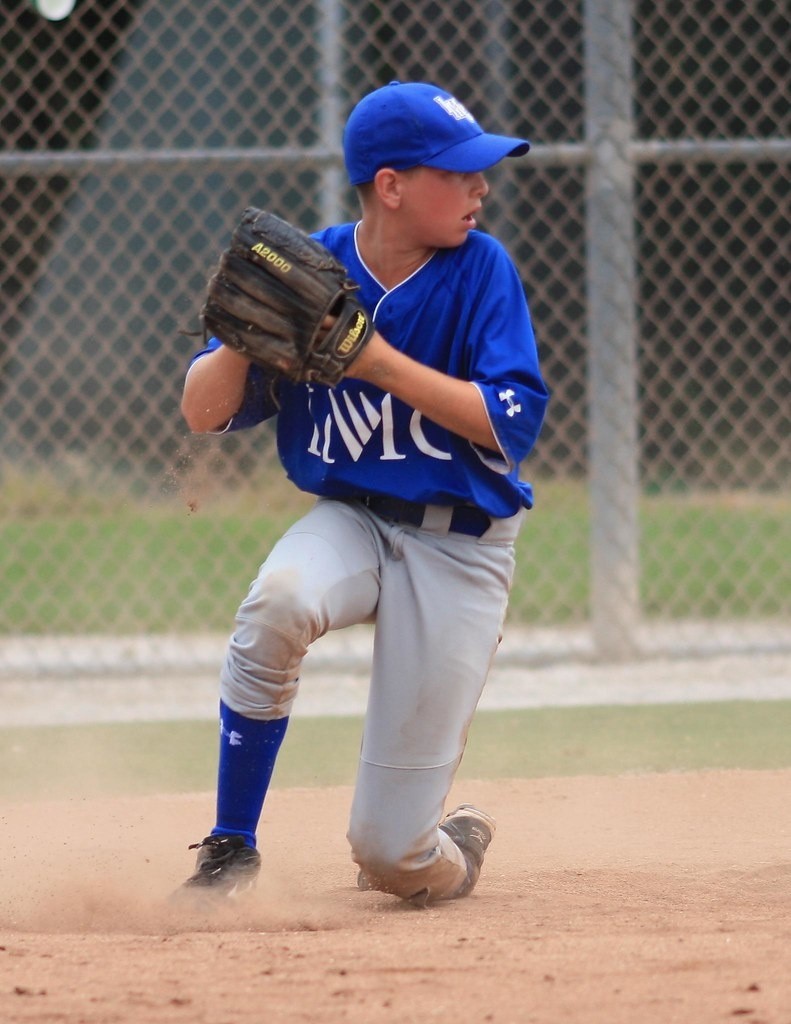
[342,80,532,187]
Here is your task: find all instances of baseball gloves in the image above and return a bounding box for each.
[202,205,374,390]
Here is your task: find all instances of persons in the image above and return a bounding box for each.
[169,81,551,910]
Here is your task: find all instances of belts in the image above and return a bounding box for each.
[354,490,490,538]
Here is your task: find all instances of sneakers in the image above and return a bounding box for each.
[441,803,498,867]
[177,833,262,909]
